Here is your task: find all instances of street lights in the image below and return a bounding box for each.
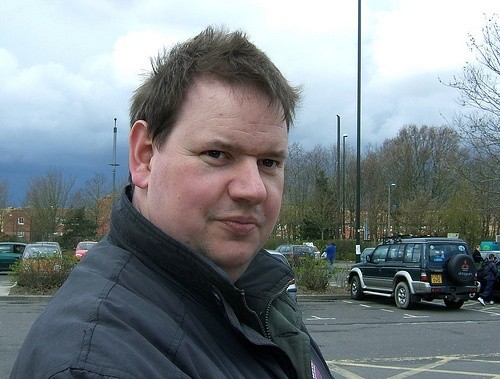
[341,133,349,240]
[387,183,397,234]
[336,114,342,235]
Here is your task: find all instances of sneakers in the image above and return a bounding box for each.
[478,297,485,306]
[490,301,494,304]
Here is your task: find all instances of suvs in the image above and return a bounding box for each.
[345,235,482,311]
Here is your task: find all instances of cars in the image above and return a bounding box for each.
[21,244,61,276]
[36,241,63,259]
[0,242,26,271]
[274,245,315,264]
[360,247,376,263]
[307,244,321,260]
[479,249,500,263]
[265,248,298,304]
[73,241,98,262]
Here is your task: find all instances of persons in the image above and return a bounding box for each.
[13,24,334,378]
[323,244,336,265]
[476,253,497,306]
[473,245,484,265]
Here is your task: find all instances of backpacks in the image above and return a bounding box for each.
[477,266,485,282]
